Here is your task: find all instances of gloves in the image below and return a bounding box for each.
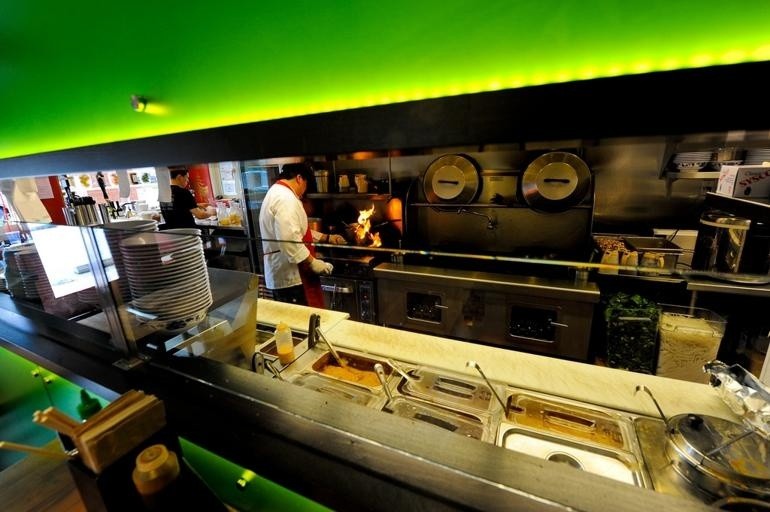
[310,258,329,274]
[327,234,347,245]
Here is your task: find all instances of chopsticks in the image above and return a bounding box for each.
[0,406,81,461]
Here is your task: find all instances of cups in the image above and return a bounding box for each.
[314,170,329,193]
[598,248,639,275]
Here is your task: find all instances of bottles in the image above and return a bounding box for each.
[131,442,176,493]
[274,319,294,365]
[338,174,350,193]
[216,200,240,226]
[116,201,123,216]
[111,201,119,219]
[77,390,102,420]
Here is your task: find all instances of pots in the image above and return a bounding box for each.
[663,413,770,511]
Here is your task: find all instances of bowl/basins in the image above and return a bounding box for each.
[138,203,148,212]
[673,147,769,172]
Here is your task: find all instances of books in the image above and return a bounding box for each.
[72,389,166,475]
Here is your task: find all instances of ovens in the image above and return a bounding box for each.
[318,276,375,324]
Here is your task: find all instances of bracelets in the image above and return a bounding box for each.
[326,234,329,244]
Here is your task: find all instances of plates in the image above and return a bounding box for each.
[0,220,213,334]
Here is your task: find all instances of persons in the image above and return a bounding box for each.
[161,168,217,228]
[259,163,347,305]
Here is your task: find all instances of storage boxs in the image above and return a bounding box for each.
[716,165,770,198]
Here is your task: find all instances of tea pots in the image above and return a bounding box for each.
[639,251,665,277]
